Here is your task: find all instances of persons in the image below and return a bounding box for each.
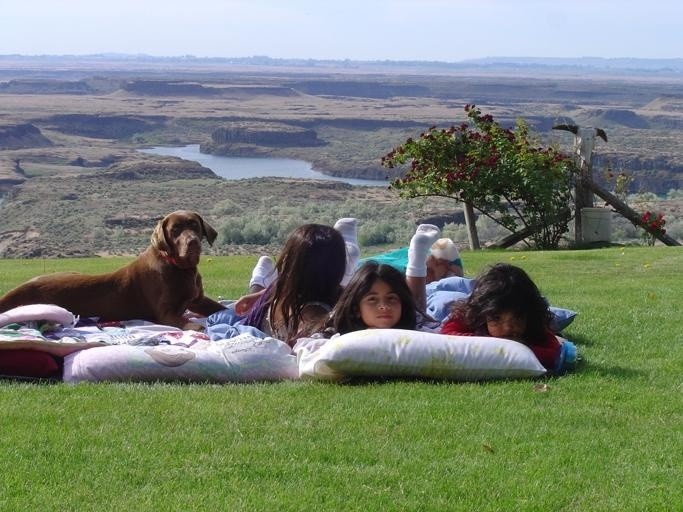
[331,217,441,335]
[234,223,348,341]
[427,238,563,366]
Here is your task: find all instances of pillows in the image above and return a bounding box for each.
[299,329,547,383]
[62,332,297,383]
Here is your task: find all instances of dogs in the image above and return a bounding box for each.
[0,210,233,327]
